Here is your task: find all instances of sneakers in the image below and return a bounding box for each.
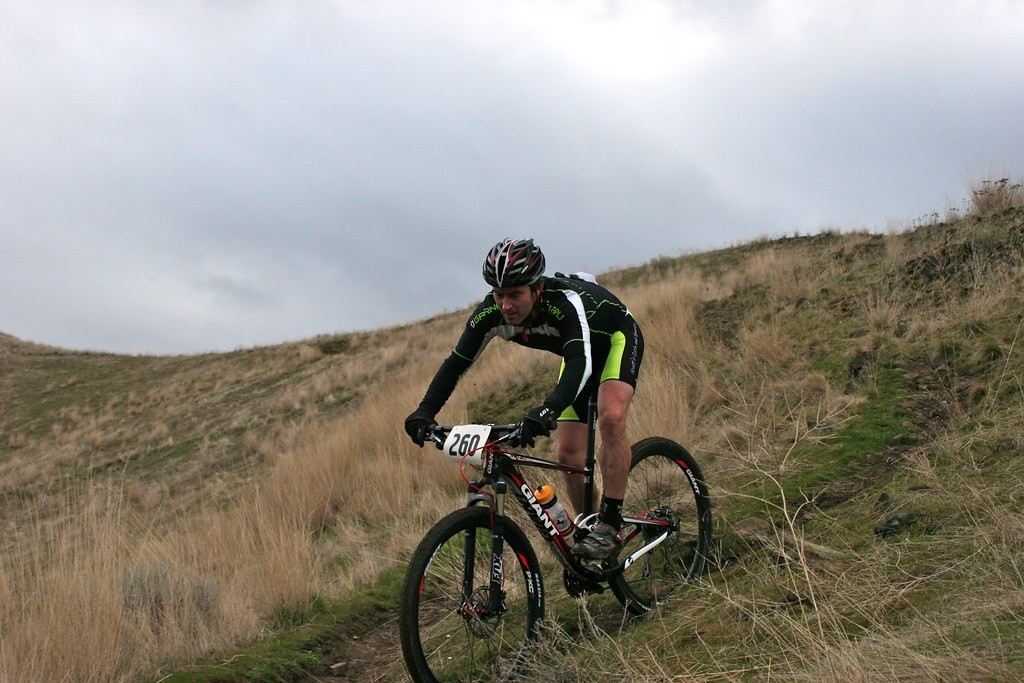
[570,521,622,559]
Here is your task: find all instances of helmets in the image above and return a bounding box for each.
[482,237,546,288]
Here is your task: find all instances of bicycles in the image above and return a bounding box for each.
[396,423,712,683]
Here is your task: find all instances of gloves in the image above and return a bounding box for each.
[404,408,439,448]
[520,406,558,448]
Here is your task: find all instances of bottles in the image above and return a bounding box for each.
[534,485,573,537]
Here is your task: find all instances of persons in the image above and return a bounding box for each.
[403,239,645,562]
